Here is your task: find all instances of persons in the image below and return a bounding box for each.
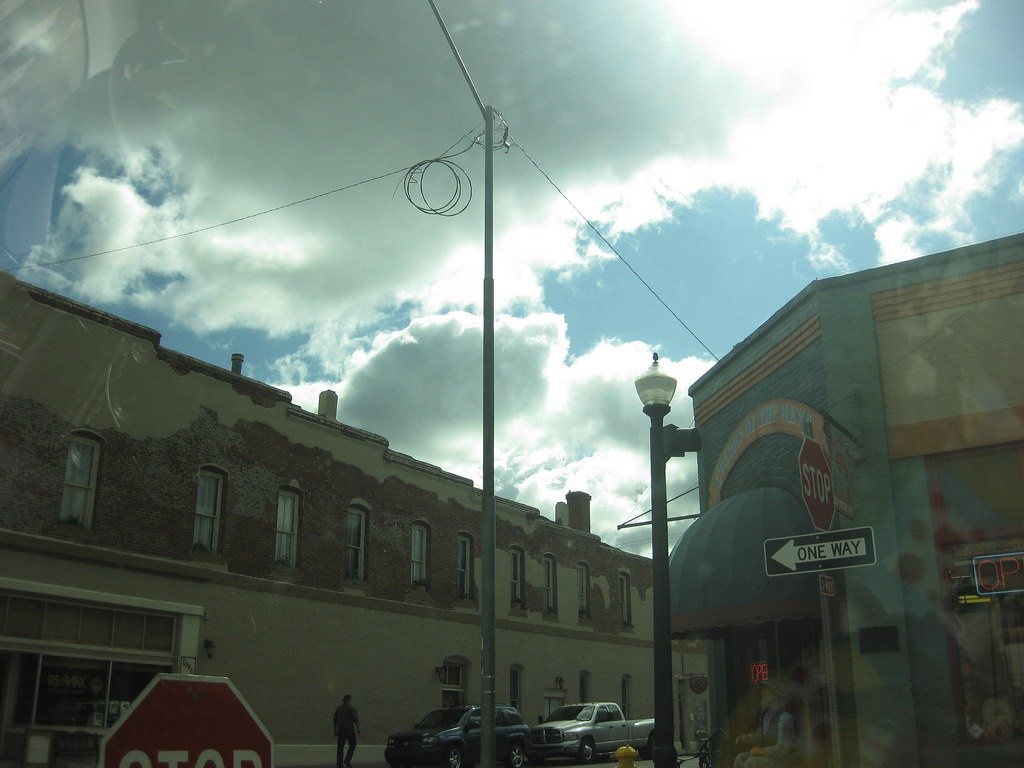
[333,694,361,768]
[733,678,796,768]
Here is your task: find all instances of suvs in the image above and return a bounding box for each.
[384,704,532,768]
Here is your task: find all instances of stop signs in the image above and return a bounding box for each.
[97,673,275,768]
[797,435,835,531]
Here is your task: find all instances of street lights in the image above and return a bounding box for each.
[635,354,701,768]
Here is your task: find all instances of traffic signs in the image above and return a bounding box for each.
[763,525,876,577]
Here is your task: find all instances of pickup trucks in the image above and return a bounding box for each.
[525,702,655,764]
